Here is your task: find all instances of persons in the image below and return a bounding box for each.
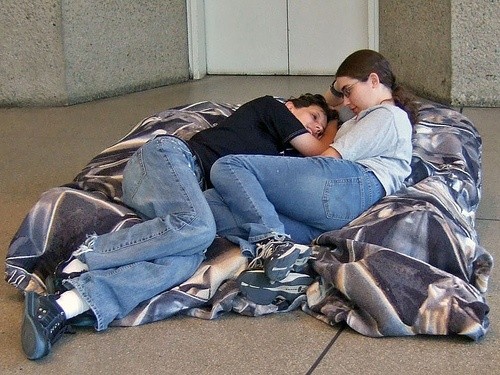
[202,50,415,305]
[20,91,342,361]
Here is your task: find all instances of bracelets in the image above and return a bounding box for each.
[328,80,343,98]
[327,108,340,123]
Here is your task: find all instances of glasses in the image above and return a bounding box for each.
[342,80,361,96]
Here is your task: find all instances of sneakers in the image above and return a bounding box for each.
[236,266,316,303]
[53,231,101,291]
[19,290,68,360]
[263,239,312,281]
